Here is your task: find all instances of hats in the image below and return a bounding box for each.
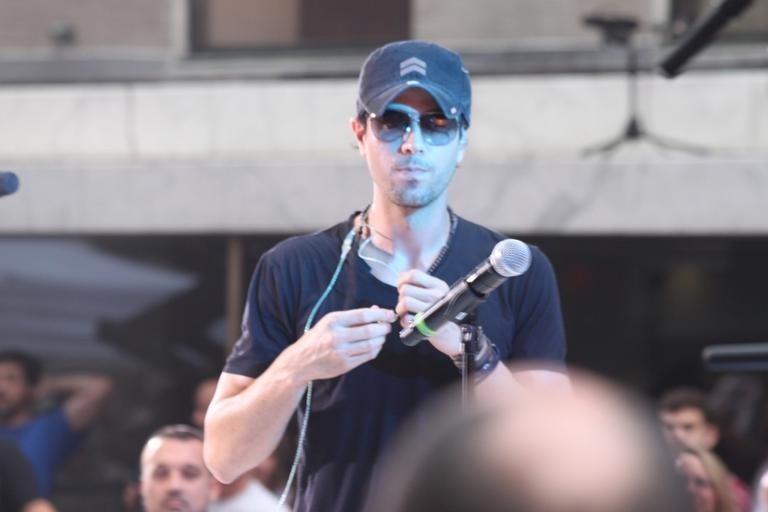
[357,41,472,127]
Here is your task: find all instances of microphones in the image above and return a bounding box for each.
[0,169,20,197]
[657,0,754,82]
[398,237,532,349]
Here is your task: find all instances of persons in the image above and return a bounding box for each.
[0,346,767,512]
[199,37,573,512]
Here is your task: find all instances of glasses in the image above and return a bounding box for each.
[371,110,458,145]
